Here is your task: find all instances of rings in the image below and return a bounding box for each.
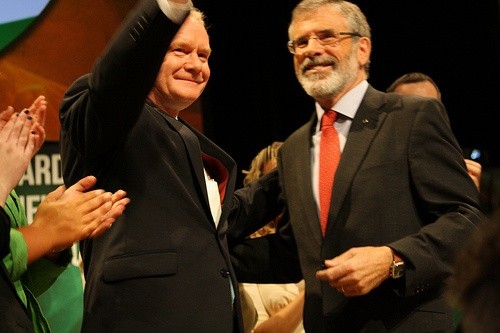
[341,285,345,293]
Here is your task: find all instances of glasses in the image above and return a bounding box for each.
[287,30,362,54]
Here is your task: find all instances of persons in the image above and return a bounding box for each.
[238,73,500,333]
[229,0,487,333]
[58,0,286,333]
[0,95,131,333]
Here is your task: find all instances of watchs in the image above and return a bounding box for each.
[390,249,405,279]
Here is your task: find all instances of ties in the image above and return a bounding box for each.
[318,110,340,235]
